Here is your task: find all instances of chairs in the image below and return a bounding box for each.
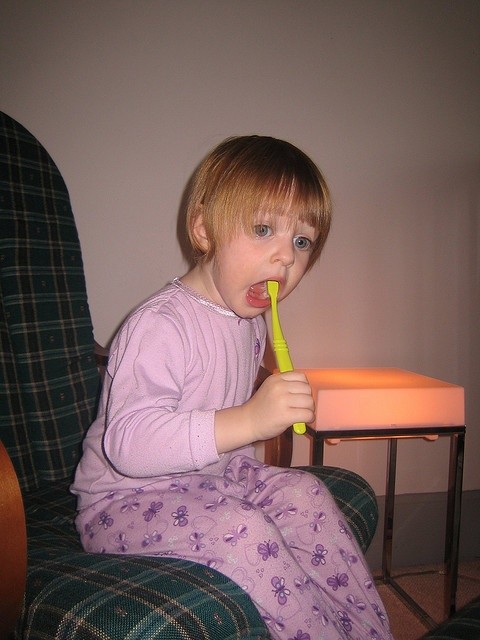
[0,111,379,640]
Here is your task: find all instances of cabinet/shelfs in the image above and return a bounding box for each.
[279,425,468,631]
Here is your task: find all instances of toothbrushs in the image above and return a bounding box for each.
[266,280,308,434]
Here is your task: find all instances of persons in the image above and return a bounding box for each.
[69,136,398,640]
